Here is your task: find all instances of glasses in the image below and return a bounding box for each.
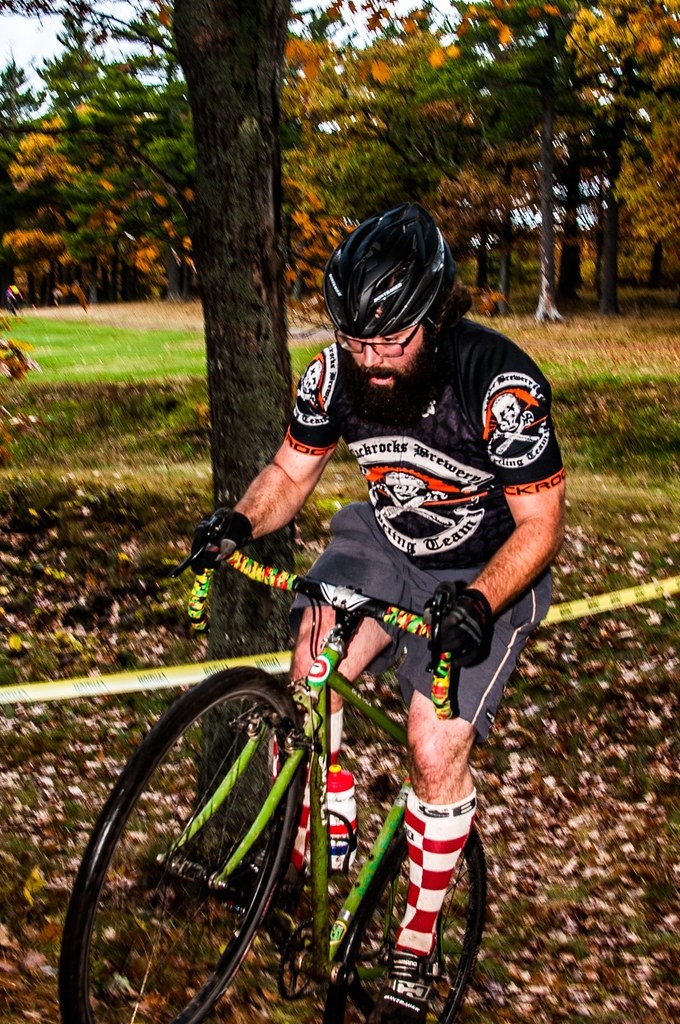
[333,321,422,358]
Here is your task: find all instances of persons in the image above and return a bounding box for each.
[192,202,567,1024]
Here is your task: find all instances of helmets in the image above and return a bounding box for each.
[323,201,457,338]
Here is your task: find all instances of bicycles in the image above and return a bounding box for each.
[58,507,488,1024]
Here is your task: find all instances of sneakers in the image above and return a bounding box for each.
[370,938,445,1024]
[208,850,303,917]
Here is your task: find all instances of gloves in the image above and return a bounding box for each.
[425,590,492,668]
[191,508,254,575]
